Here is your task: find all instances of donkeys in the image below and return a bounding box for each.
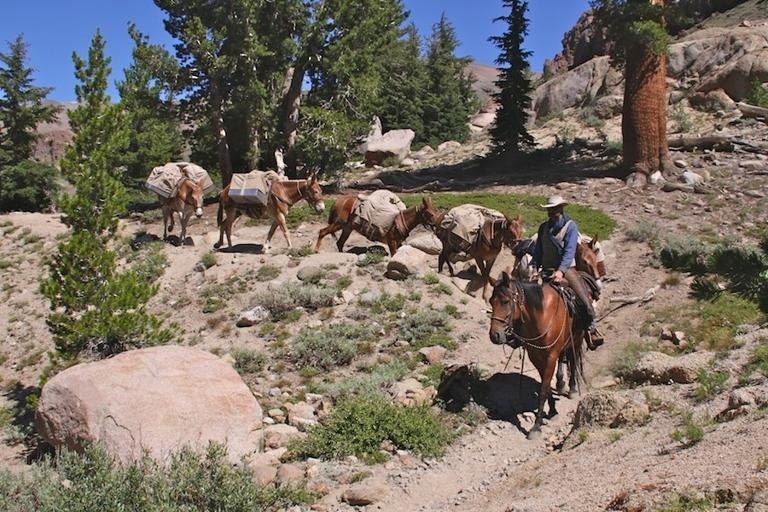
[214,172,325,251]
[434,209,521,284]
[158,176,205,245]
[511,234,629,290]
[315,195,439,258]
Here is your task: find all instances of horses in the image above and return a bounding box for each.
[485,270,586,440]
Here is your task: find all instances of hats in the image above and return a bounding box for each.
[539,194,571,211]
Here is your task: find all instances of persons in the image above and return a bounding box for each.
[525,196,604,346]
[274,144,288,176]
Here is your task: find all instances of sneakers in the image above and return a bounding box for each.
[586,332,605,347]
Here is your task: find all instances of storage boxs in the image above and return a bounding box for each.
[225,172,271,206]
[143,166,179,199]
[576,238,608,281]
[187,166,213,191]
